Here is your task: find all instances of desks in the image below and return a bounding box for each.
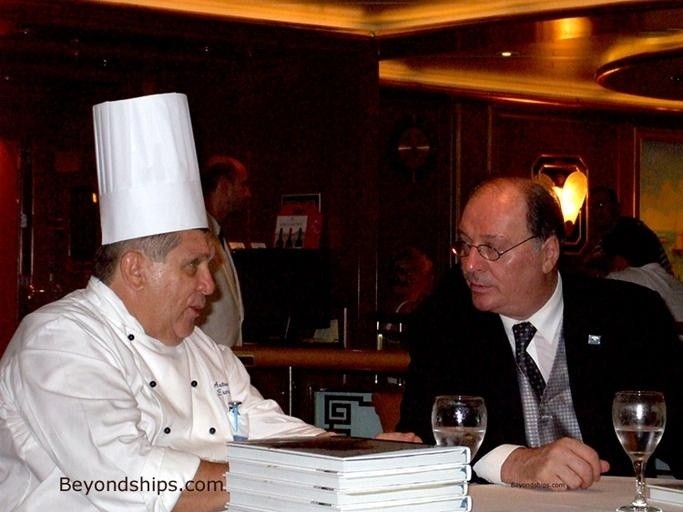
[464,474,682,512]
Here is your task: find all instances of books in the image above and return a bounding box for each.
[221,434,475,512]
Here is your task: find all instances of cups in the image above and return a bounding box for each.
[430,395,489,463]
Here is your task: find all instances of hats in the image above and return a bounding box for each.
[92,93,210,245]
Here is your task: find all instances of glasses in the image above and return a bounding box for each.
[451,235,537,261]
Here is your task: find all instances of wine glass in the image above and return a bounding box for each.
[610,388,668,512]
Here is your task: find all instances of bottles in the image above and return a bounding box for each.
[274,226,304,249]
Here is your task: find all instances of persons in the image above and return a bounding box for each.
[190,154,255,349]
[380,176,681,511]
[0,222,425,511]
[275,226,303,249]
